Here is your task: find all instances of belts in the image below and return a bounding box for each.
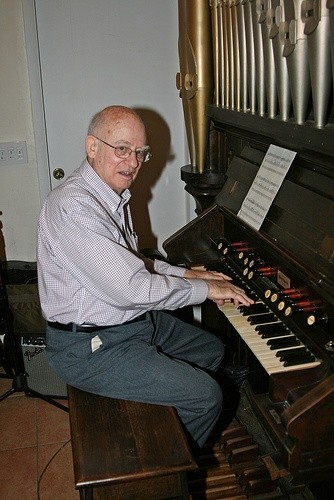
[48,312,147,333]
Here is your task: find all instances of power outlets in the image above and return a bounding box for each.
[0,141,29,166]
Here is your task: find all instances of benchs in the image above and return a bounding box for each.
[67,384,199,500]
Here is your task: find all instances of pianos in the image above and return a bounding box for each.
[161,103,334,500]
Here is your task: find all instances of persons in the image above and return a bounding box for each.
[37,105,254,465]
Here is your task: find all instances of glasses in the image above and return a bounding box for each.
[92,134,152,164]
